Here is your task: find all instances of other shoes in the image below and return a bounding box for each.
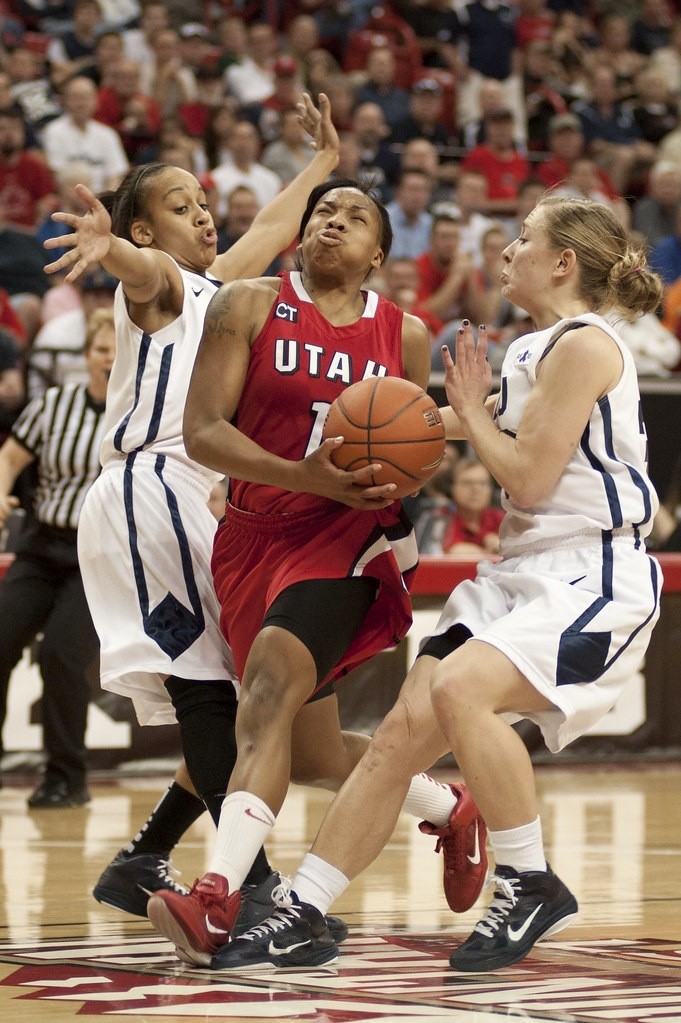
[27,772,91,809]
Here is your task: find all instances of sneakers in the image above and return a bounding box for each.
[419,781,489,913]
[209,883,340,971]
[147,870,249,967]
[230,872,347,945]
[92,848,190,917]
[449,854,579,970]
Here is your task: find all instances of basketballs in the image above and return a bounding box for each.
[322,376,446,500]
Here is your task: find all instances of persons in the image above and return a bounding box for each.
[43,93,349,944]
[212,194,663,972]
[0,0,681,806]
[147,178,490,965]
